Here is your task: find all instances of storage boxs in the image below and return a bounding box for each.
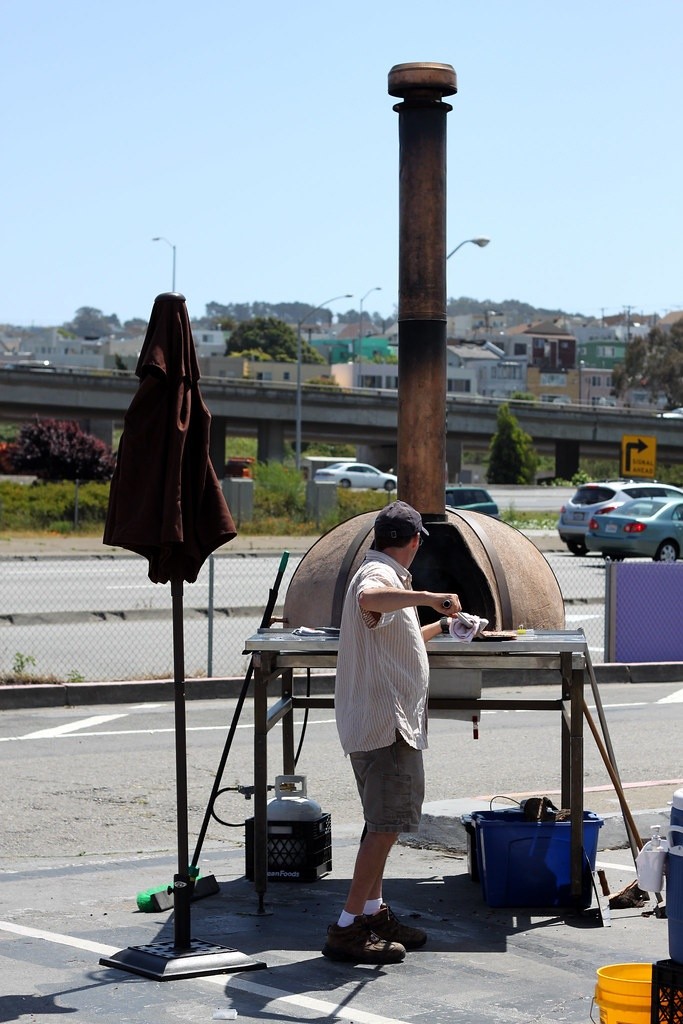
[246,813,335,885]
[462,810,603,909]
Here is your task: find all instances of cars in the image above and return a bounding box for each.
[315,462,398,492]
[586,496,682,561]
[657,407,683,419]
[446,487,499,519]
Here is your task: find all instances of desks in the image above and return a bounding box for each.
[242,627,592,919]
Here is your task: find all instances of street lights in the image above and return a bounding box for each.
[296,295,354,469]
[359,288,381,387]
[153,237,176,293]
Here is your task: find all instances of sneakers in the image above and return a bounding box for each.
[321,915,406,964]
[364,902,428,948]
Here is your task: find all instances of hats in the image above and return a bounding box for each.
[374,499,429,540]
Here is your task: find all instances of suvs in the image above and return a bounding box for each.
[557,478,682,557]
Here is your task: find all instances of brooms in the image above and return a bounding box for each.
[135,551,292,913]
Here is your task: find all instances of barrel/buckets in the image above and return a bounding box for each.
[590,963,651,1024]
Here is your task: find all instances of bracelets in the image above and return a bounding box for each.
[440,616,449,634]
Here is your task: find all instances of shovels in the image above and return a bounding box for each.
[442,601,517,640]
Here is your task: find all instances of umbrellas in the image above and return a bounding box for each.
[101,292,267,980]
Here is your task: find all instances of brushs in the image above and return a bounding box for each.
[137,551,291,913]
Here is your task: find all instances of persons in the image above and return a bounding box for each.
[321,500,489,970]
